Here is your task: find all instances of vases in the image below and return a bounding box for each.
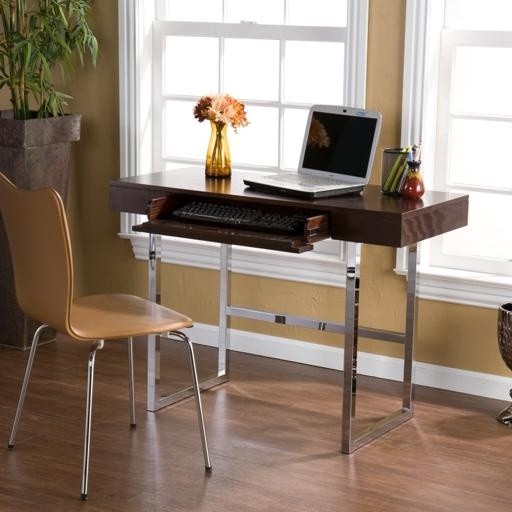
[205,120,232,178]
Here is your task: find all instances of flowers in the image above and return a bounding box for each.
[193,94,250,167]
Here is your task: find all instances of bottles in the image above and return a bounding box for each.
[401,161,424,198]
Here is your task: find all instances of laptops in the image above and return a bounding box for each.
[243,104,382,198]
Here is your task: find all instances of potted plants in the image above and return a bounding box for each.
[0,0,99,352]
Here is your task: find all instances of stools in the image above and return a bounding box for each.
[497,303,512,428]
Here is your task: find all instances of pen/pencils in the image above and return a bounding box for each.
[382,146,413,194]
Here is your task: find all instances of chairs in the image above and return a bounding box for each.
[0,171,212,502]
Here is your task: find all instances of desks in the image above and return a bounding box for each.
[110,166,469,454]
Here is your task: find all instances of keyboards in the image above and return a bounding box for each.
[172,201,309,235]
